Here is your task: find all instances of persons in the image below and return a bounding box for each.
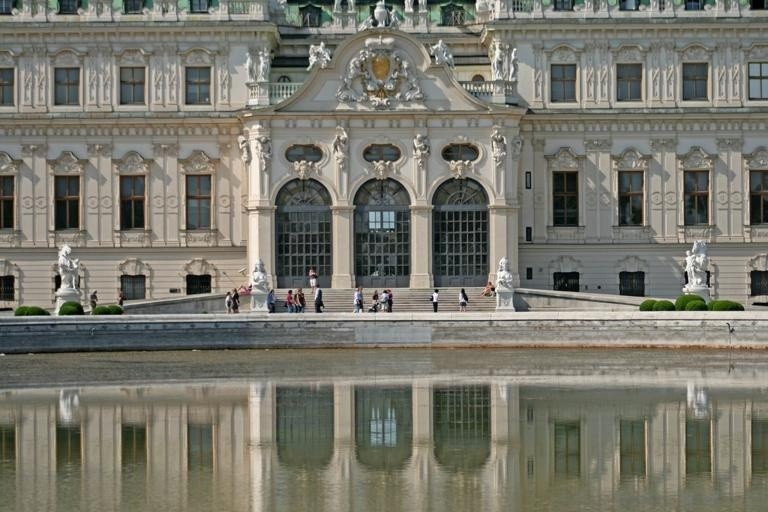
[314,284,324,312]
[251,260,268,289]
[309,269,318,293]
[481,282,494,297]
[497,257,514,288]
[287,288,305,312]
[353,286,363,312]
[372,289,393,312]
[90,290,98,310]
[432,289,439,312]
[58,391,80,424]
[238,39,522,170]
[459,289,468,312]
[226,288,239,313]
[61,253,80,289]
[266,289,275,312]
[685,382,699,411]
[118,288,124,306]
[684,249,698,285]
